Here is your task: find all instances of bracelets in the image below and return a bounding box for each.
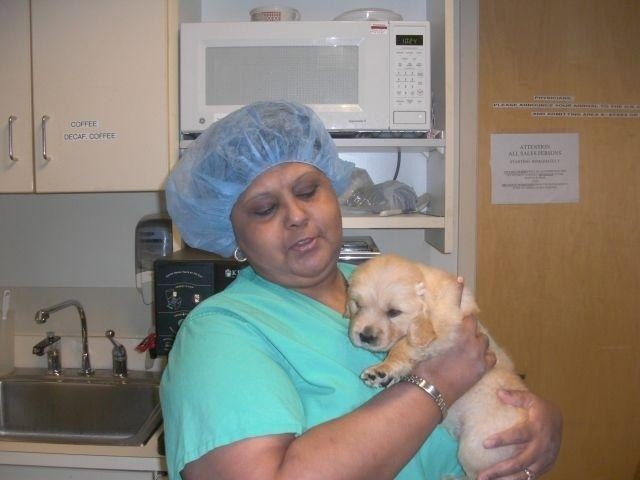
[400,373,448,424]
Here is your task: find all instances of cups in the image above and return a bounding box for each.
[247,6,300,21]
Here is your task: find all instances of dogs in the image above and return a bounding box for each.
[343,254,530,480]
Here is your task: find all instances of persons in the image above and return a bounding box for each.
[158,102,563,479]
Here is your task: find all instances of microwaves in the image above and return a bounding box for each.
[179,21,434,140]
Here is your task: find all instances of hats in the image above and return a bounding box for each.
[163,99,356,259]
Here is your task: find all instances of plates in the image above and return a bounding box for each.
[333,9,401,21]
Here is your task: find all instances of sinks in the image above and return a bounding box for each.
[0,367,163,448]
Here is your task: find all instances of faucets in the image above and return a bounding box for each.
[35,300,95,376]
[32,332,64,376]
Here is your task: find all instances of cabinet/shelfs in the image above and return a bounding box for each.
[2,438,167,480]
[172,0,456,254]
[1,1,179,191]
